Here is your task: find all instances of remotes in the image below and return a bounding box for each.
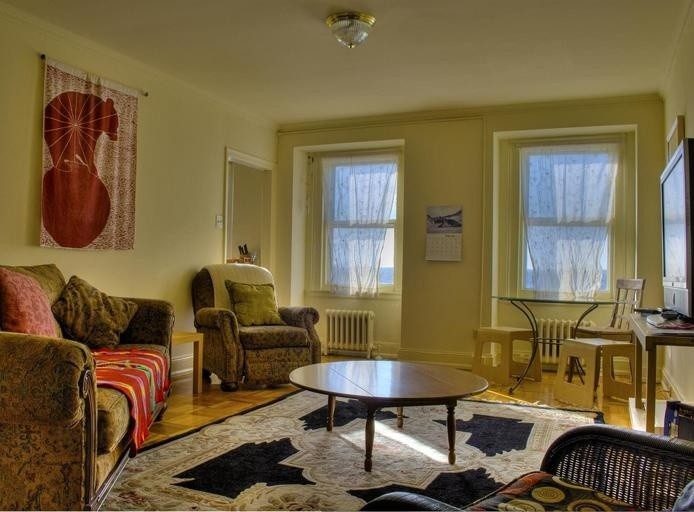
[660,307,680,321]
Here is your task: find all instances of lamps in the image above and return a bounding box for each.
[324,8,378,52]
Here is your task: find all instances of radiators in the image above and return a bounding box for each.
[532,318,591,366]
[323,309,376,359]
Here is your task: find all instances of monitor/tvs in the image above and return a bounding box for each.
[658,137,694,326]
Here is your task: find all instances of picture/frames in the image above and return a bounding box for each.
[662,114,686,163]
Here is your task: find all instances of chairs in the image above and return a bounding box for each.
[552,277,647,392]
[354,423,694,511]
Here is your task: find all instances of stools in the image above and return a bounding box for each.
[553,337,636,409]
[471,326,542,386]
[169,332,205,395]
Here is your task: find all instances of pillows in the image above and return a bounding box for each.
[0,265,58,337]
[52,274,138,350]
[672,478,694,511]
[224,278,288,326]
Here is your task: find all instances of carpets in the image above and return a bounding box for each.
[98,387,607,512]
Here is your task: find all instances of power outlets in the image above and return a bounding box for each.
[665,386,672,397]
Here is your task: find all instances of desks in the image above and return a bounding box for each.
[627,309,693,434]
[492,296,618,394]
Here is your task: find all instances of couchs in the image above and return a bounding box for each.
[0,263,175,512]
[190,263,323,393]
